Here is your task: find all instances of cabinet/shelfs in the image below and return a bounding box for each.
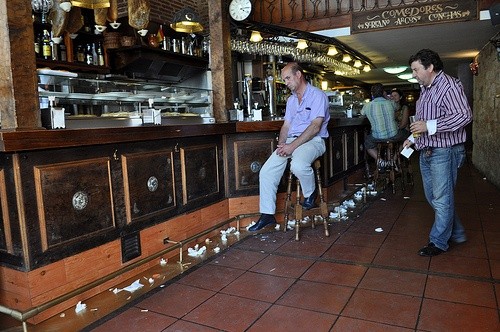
[263,62,315,106]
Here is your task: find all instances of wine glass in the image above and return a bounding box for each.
[230,38,361,76]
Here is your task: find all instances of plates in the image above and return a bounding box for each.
[39,91,211,103]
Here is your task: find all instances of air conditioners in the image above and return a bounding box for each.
[296,40,308,50]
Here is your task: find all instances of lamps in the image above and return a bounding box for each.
[249,31,263,43]
[170,7,204,34]
[363,65,372,73]
[327,47,339,56]
[383,66,419,84]
[353,60,363,67]
[342,54,352,63]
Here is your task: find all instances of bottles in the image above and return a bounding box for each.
[264,64,290,118]
[34,30,104,66]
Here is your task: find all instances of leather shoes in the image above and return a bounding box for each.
[418,242,444,257]
[249,214,276,231]
[303,189,318,210]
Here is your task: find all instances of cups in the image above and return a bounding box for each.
[148,33,209,57]
[409,116,422,138]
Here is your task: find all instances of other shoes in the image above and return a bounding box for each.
[378,162,392,174]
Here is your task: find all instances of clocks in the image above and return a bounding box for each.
[226,0,253,27]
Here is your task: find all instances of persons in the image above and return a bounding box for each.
[389,88,410,131]
[360,83,412,172]
[248,64,330,232]
[402,49,473,256]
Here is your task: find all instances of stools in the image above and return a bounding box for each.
[284,153,330,240]
[373,138,414,196]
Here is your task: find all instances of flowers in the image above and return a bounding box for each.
[468,62,479,75]
